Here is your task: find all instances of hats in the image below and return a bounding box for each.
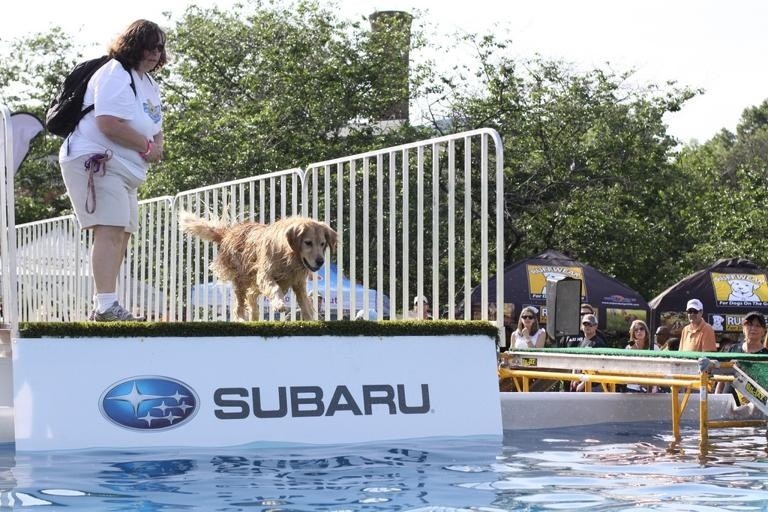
[685,299,703,312]
[582,313,598,326]
[744,310,766,328]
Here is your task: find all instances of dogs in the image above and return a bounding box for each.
[176,199,339,323]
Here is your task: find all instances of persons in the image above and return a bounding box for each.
[567,314,608,393]
[411,295,432,320]
[625,319,739,393]
[57,18,168,325]
[677,299,718,352]
[714,311,768,394]
[559,304,608,348]
[509,305,547,351]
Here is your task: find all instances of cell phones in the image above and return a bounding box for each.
[628,341,634,346]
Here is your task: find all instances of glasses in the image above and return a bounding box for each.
[521,315,533,319]
[151,43,163,52]
[687,309,698,314]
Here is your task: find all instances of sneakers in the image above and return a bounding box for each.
[93,302,145,323]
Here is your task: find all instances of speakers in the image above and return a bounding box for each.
[545,272,582,340]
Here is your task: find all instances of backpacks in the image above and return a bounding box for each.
[44,54,136,137]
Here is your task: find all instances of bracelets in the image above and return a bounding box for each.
[139,141,152,156]
[525,338,532,343]
